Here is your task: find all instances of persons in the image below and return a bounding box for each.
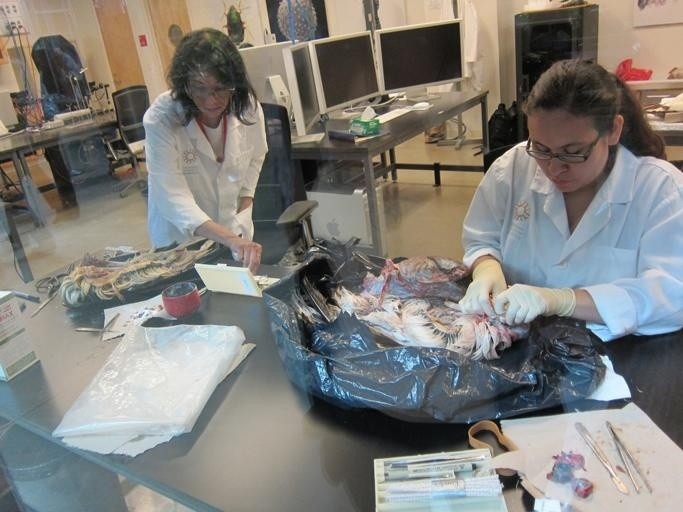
[456,57,683,343]
[143,27,268,276]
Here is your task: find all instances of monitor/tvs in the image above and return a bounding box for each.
[375,17,466,97]
[310,29,383,117]
[237,39,295,121]
[282,42,326,144]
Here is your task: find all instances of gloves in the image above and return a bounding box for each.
[495,282,576,326]
[458,259,508,316]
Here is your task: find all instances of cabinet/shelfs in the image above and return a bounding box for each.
[515,4,599,144]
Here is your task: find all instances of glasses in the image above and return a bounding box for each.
[182,81,240,101]
[526,134,601,163]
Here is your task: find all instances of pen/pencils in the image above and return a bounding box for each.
[75,314,121,335]
[384,461,477,481]
[13,291,40,302]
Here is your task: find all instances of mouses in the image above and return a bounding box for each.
[411,101,430,110]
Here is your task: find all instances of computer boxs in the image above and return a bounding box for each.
[304,180,386,251]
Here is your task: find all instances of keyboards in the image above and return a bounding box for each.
[376,107,412,125]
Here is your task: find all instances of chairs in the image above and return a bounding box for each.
[100,85,151,198]
[249,102,319,265]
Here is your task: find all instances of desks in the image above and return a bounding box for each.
[290,89,490,259]
[623,79,683,113]
[0,247,683,512]
[0,109,118,284]
